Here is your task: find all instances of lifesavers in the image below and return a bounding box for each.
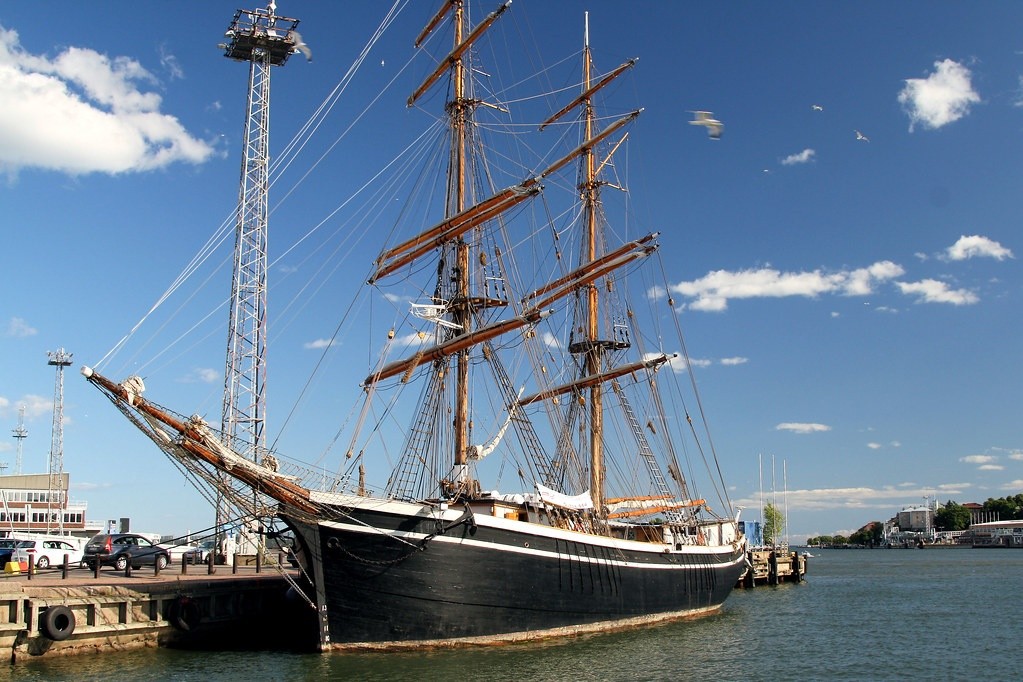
[697,532,706,545]
[42,605,76,641]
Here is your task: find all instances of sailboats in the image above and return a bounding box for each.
[77,0,754,654]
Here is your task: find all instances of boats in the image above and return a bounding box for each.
[801,550,815,558]
[887,536,974,549]
[811,540,866,549]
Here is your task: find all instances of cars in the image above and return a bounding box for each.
[0,537,84,569]
[81,534,171,570]
[182,540,221,564]
